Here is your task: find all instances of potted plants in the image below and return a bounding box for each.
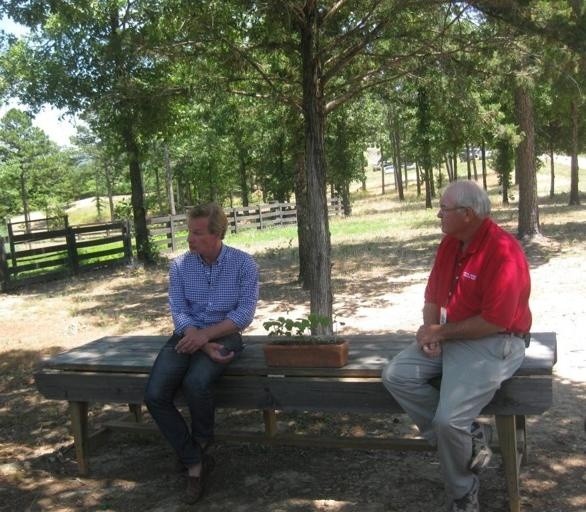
[259,307,352,366]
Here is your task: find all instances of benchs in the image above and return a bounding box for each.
[32,330,559,512]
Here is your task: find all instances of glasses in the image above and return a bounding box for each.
[437,203,465,215]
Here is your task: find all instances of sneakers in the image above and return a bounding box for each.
[181,454,208,505]
[450,478,481,512]
[467,422,492,475]
[175,429,215,473]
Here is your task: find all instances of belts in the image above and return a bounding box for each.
[499,331,524,340]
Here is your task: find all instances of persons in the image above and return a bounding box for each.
[382,182,532,512]
[146,201,260,504]
